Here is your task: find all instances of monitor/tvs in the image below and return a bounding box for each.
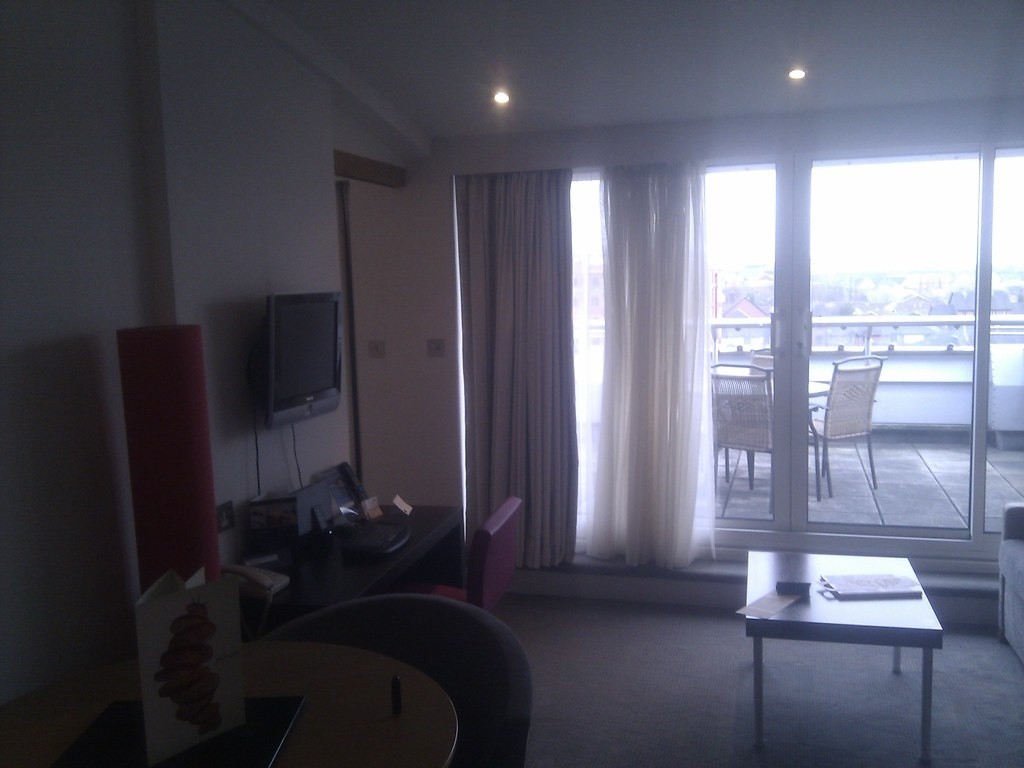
[260,288,347,432]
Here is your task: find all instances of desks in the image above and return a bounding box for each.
[222,504,467,642]
[743,555,943,768]
[747,379,828,490]
[0,642,458,767]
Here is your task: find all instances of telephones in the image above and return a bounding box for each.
[223,562,291,598]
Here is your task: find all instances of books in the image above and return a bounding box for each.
[822,574,922,599]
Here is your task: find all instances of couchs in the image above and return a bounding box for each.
[997,500,1024,668]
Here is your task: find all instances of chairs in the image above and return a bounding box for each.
[704,361,776,513]
[258,593,529,768]
[806,353,887,497]
[383,495,524,613]
